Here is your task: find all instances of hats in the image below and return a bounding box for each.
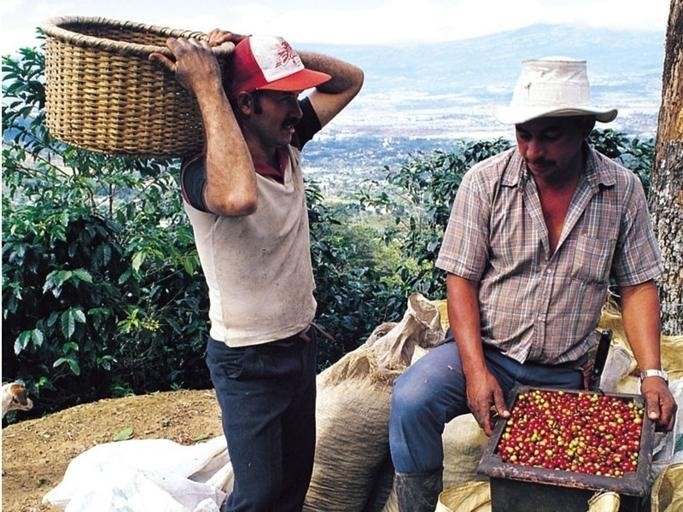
[226,32,333,100]
[496,57,618,126]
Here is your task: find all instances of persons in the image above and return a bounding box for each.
[147,28,366,512]
[385,55,679,512]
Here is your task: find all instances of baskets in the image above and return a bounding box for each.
[38,15,238,160]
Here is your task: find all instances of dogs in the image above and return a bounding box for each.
[2,381,34,477]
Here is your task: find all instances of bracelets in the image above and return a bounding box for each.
[637,369,669,386]
